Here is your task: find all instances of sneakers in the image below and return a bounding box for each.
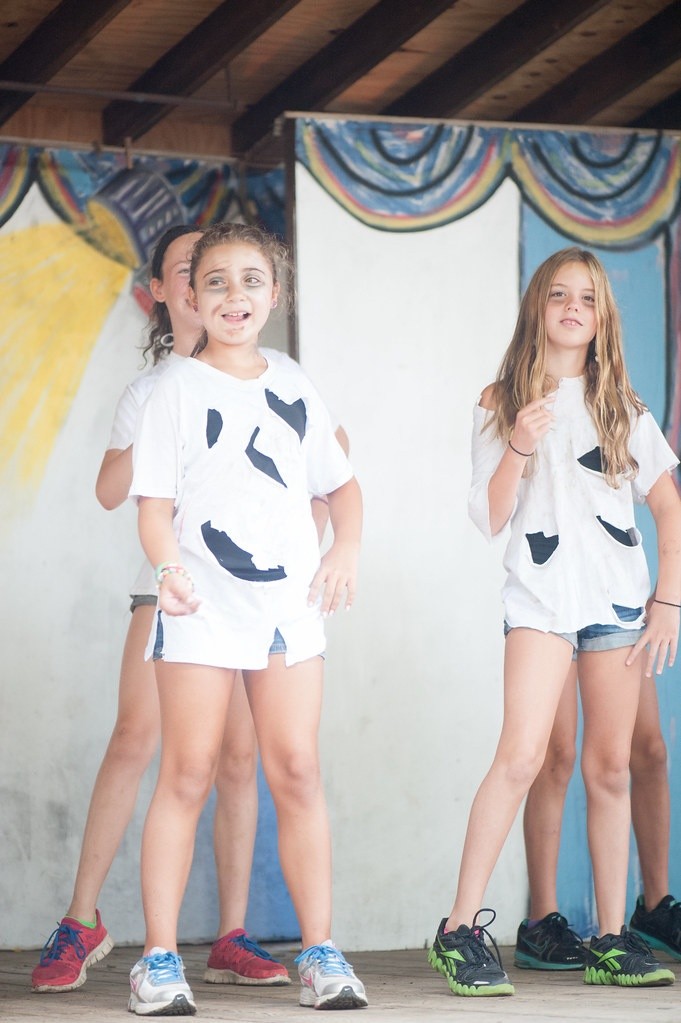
[126,946,197,1015]
[202,928,291,985]
[513,912,588,970]
[426,908,515,996]
[581,925,675,986]
[293,939,368,1009]
[30,908,114,992]
[628,894,680,961]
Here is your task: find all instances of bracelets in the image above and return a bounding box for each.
[653,600,681,608]
[508,440,534,456]
[154,561,192,584]
[311,497,330,506]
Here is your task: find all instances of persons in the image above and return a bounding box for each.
[30,225,353,995]
[124,223,374,1018]
[512,646,681,972]
[426,246,681,999]
